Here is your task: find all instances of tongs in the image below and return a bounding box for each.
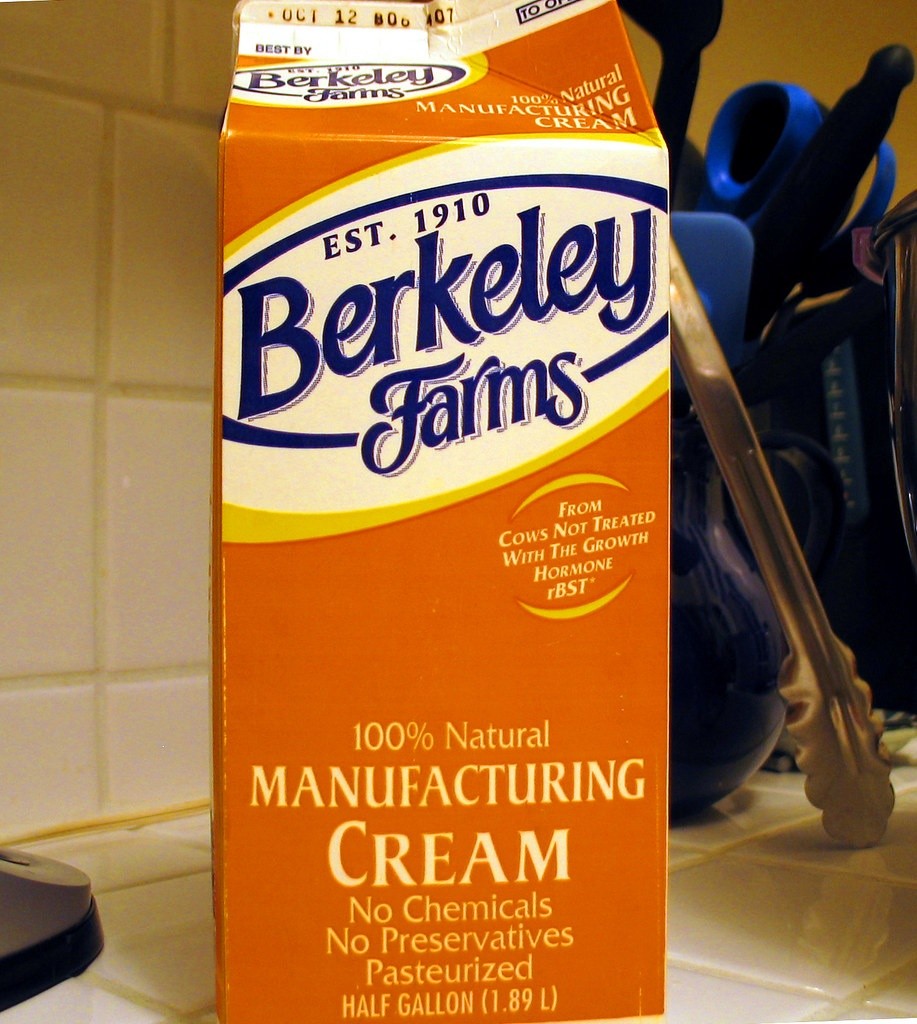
[668,226,897,851]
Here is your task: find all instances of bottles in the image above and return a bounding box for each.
[671,207,788,820]
